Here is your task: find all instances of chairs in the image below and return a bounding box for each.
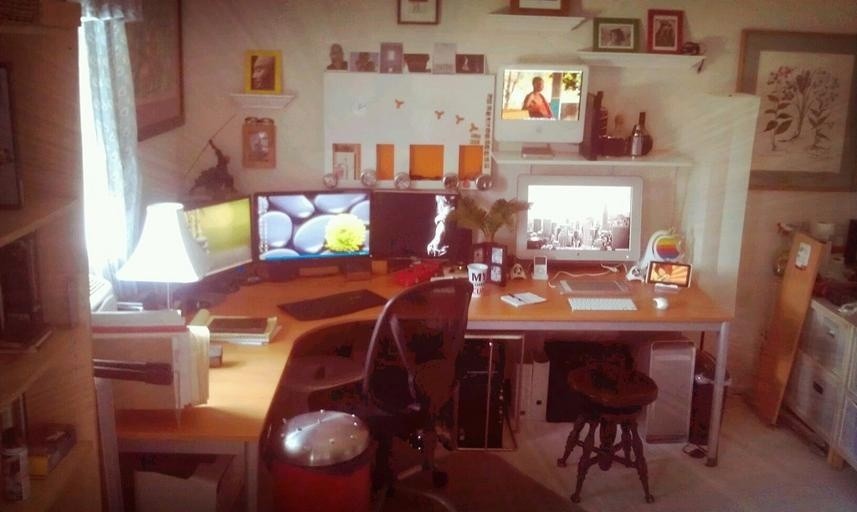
[315,278,474,511]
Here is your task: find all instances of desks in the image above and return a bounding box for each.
[90,257,731,511]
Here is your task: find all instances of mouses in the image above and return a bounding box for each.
[651,296,668,310]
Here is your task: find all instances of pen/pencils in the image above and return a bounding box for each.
[508,292,528,304]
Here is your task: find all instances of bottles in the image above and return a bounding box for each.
[630,124,643,160]
[0,427,29,504]
[608,113,629,155]
[629,110,653,157]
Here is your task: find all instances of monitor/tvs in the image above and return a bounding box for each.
[493,62,589,159]
[373,188,459,273]
[184,194,254,293]
[516,174,644,274]
[253,189,373,265]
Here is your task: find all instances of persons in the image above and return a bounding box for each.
[605,28,626,47]
[327,43,347,71]
[521,77,553,119]
[655,263,672,283]
[252,56,274,89]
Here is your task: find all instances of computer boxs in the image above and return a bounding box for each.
[458,348,505,448]
[633,334,697,445]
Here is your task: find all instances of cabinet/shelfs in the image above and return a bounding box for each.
[0,1,102,512]
[780,293,857,471]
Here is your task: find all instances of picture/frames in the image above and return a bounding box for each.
[647,10,683,54]
[243,49,283,95]
[241,124,276,168]
[510,0,571,16]
[122,1,186,143]
[397,0,437,25]
[735,29,856,192]
[591,18,638,52]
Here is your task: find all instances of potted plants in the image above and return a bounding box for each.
[445,194,532,286]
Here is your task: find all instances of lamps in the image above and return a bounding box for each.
[112,202,200,310]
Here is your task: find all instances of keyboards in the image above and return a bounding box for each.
[568,297,637,311]
[278,289,388,321]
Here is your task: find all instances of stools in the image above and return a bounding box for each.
[550,359,659,504]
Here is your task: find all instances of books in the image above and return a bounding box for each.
[0,230,53,354]
[24,423,77,480]
[188,309,281,346]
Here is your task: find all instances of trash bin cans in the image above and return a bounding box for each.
[692,354,730,446]
[267,410,378,512]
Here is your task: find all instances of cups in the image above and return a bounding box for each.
[807,220,835,242]
[466,263,488,299]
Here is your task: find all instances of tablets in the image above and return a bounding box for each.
[559,280,630,294]
[645,260,693,288]
